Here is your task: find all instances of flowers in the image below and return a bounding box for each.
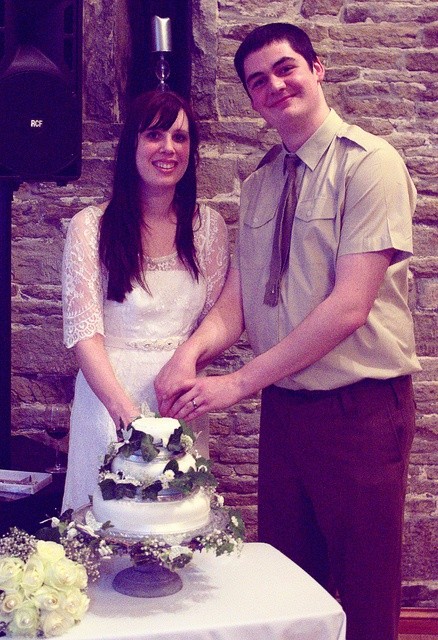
[0,530,91,638]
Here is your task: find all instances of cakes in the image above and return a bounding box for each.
[93,416,213,534]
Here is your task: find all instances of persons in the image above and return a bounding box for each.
[61,91,231,513]
[154,22,418,638]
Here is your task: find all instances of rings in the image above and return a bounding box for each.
[192,401,197,408]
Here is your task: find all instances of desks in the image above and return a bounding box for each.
[0,433,69,530]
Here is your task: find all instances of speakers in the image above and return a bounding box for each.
[0,0,83,187]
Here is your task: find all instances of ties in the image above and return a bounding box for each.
[264,153,302,308]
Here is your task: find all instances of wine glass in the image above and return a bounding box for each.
[44,404,71,475]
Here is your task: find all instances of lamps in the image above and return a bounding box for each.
[152,16,173,90]
